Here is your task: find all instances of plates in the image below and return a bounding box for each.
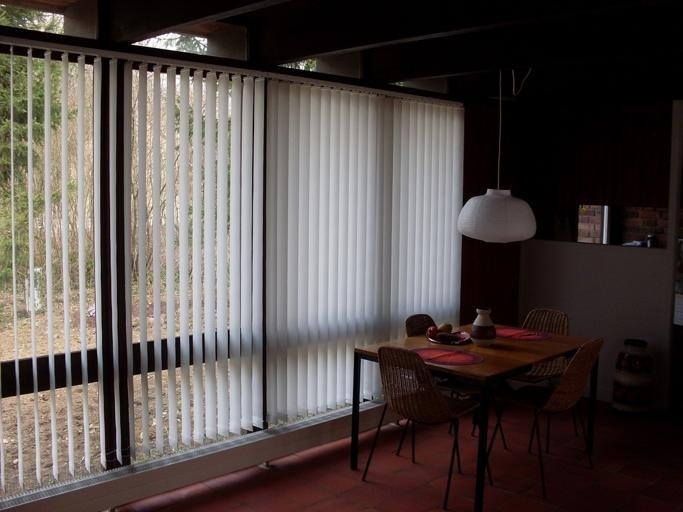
[424,329,470,346]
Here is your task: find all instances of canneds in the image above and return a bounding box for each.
[647,235,655,248]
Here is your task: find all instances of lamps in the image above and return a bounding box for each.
[456,58,537,246]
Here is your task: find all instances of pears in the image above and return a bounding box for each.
[437,322,453,333]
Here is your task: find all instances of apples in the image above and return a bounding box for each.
[425,326,437,338]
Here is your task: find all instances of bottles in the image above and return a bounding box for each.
[646,234,654,247]
[469,307,496,346]
